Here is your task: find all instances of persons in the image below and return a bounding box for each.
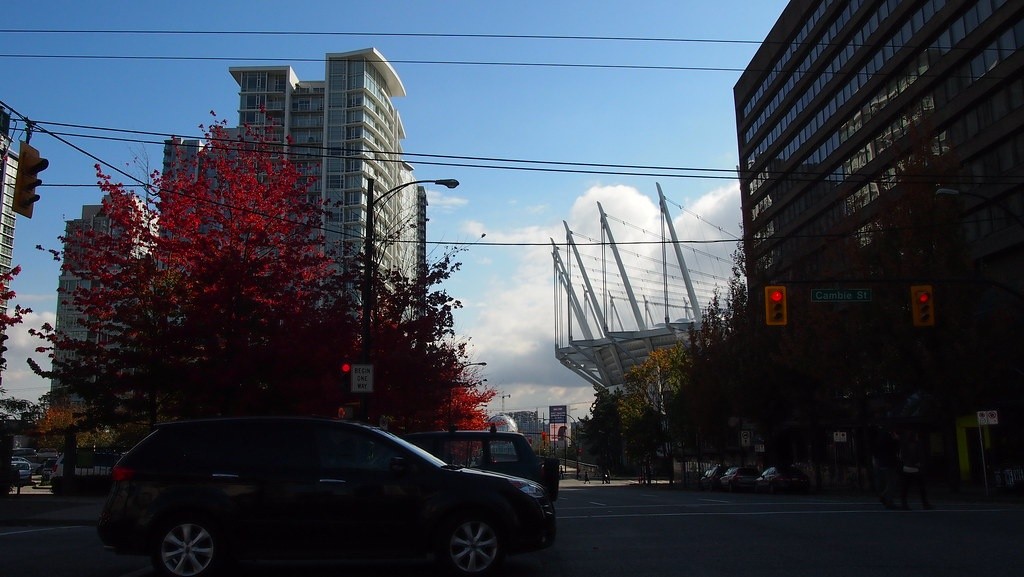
[559,464,611,484]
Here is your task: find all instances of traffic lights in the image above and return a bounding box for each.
[764,285,787,325]
[11,140,49,219]
[910,285,936,327]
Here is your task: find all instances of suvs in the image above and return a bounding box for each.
[94,413,558,577]
[395,428,560,501]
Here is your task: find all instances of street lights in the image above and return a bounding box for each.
[360,171,459,425]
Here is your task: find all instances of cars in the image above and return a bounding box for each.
[8,446,122,487]
[697,461,811,497]
